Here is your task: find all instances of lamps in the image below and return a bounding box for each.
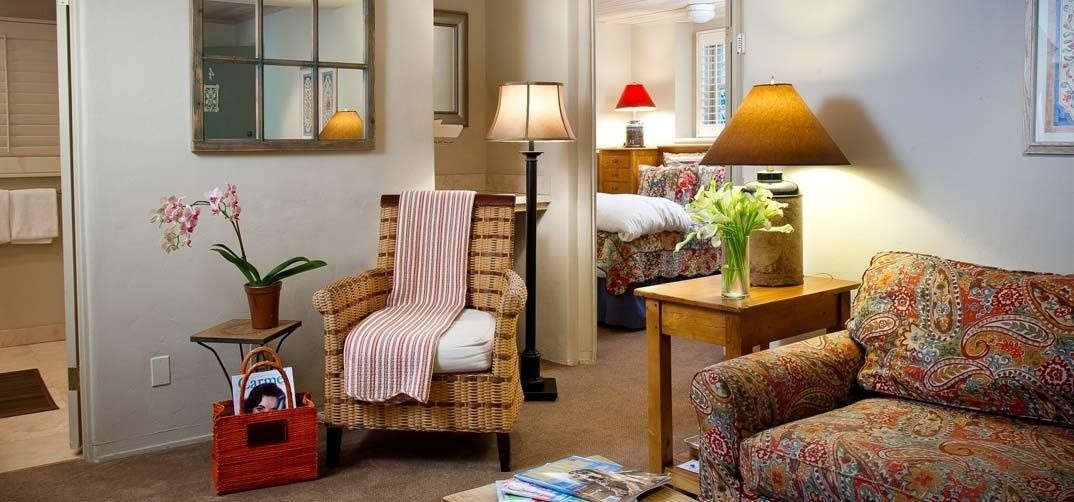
[682,1,716,23]
[318,104,364,140]
[612,81,656,148]
[693,74,852,288]
[483,81,581,405]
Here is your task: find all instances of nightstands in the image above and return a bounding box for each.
[597,147,662,194]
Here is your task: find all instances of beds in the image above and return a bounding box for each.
[596,145,729,337]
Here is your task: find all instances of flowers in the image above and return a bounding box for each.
[146,178,329,286]
[671,180,796,294]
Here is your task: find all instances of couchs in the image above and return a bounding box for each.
[687,246,1072,501]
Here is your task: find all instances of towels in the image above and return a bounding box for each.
[0,187,10,248]
[9,188,62,247]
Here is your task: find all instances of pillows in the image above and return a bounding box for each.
[664,157,725,194]
[633,162,698,207]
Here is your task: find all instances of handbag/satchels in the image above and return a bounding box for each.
[209,347,320,495]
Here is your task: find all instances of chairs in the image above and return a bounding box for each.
[307,191,529,475]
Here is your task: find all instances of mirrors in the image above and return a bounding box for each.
[435,8,473,133]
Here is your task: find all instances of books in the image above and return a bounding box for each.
[496,454,675,502]
[231,366,297,415]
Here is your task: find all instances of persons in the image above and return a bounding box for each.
[244,383,286,413]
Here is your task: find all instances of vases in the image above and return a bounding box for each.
[241,281,283,332]
[716,236,751,299]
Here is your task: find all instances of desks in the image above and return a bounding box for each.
[630,270,860,502]
[185,317,304,398]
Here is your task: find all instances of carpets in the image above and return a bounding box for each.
[0,364,60,419]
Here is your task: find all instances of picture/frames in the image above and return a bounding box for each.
[318,66,341,132]
[299,66,313,140]
[1018,0,1073,158]
